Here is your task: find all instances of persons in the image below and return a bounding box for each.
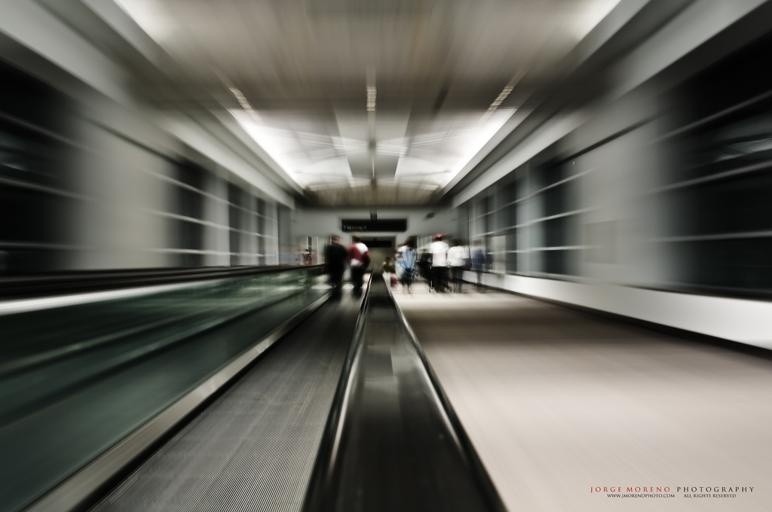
[325,234,347,301]
[383,233,486,294]
[346,236,371,297]
